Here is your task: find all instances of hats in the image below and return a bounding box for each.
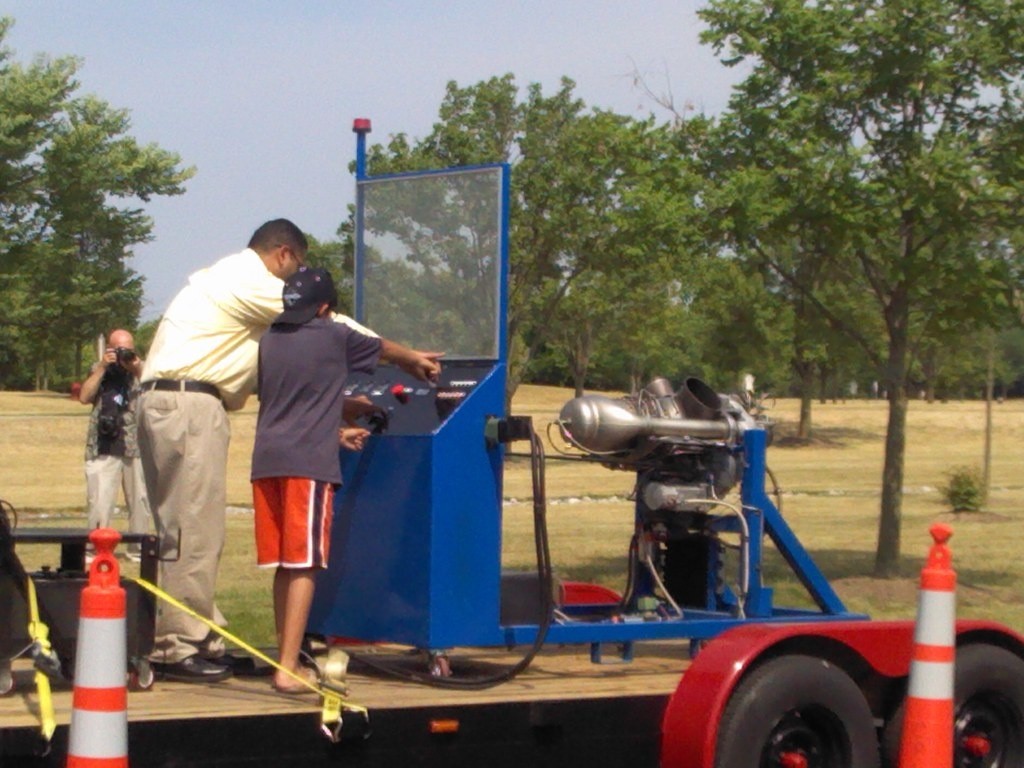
[270,268,336,328]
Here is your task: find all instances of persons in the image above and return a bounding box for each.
[250,266,441,695]
[79,328,154,566]
[133,217,445,684]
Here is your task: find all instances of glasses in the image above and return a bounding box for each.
[275,244,304,270]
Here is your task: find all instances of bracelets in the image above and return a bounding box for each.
[339,429,344,440]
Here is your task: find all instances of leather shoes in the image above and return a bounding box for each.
[148,653,233,683]
[204,654,255,675]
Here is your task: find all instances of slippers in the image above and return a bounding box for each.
[276,666,319,693]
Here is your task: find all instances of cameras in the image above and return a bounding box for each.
[113,347,135,362]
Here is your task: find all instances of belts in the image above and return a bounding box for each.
[142,380,221,400]
